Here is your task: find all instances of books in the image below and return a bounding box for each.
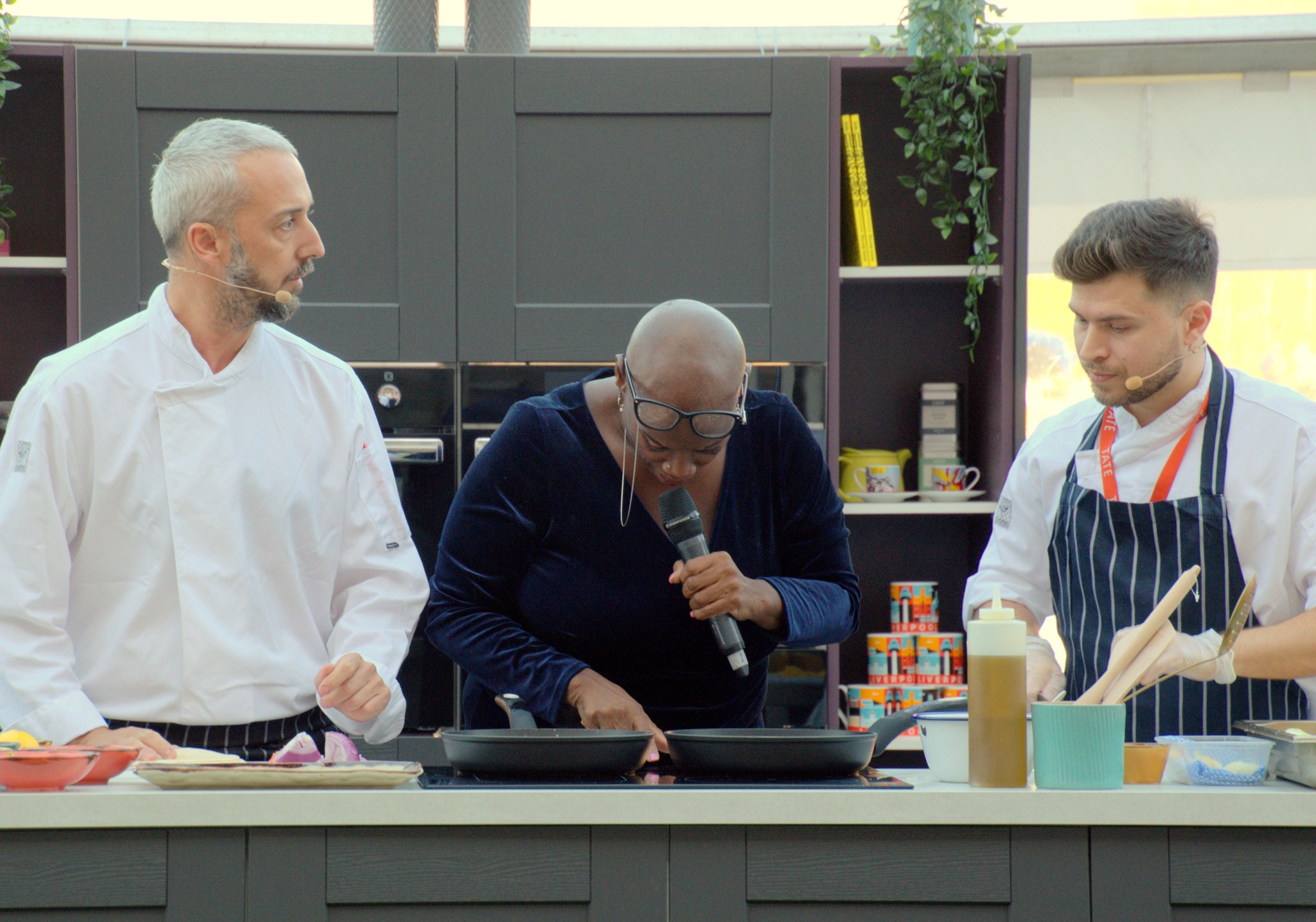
[836,113,879,268]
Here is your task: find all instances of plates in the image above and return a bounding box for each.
[848,490,920,503]
[134,760,427,788]
[922,489,986,501]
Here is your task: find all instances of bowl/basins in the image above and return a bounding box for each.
[912,709,1037,784]
[45,746,139,786]
[1123,743,1170,784]
[0,749,98,790]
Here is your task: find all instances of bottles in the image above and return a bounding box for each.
[967,584,1030,789]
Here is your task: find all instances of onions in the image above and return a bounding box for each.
[273,731,321,763]
[324,731,360,763]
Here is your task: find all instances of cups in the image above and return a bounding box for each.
[1031,698,1126,790]
[930,466,980,492]
[854,465,903,495]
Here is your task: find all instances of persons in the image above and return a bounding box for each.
[0,115,430,770]
[963,197,1316,743]
[432,297,862,768]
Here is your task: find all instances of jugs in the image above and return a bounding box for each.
[838,445,912,503]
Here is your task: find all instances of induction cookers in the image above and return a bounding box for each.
[417,757,914,792]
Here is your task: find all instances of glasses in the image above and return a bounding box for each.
[624,358,748,438]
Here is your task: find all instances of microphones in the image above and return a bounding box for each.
[659,486,749,677]
[1124,352,1193,390]
[196,272,293,303]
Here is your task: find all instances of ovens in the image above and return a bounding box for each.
[341,357,844,738]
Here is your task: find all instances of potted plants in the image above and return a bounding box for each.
[854,0,1030,372]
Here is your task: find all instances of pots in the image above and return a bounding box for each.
[441,692,656,772]
[661,695,968,781]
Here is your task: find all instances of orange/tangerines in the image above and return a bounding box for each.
[0,729,39,749]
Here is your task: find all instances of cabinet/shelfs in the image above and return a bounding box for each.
[76,43,835,368]
[836,44,1036,738]
[0,34,81,439]
